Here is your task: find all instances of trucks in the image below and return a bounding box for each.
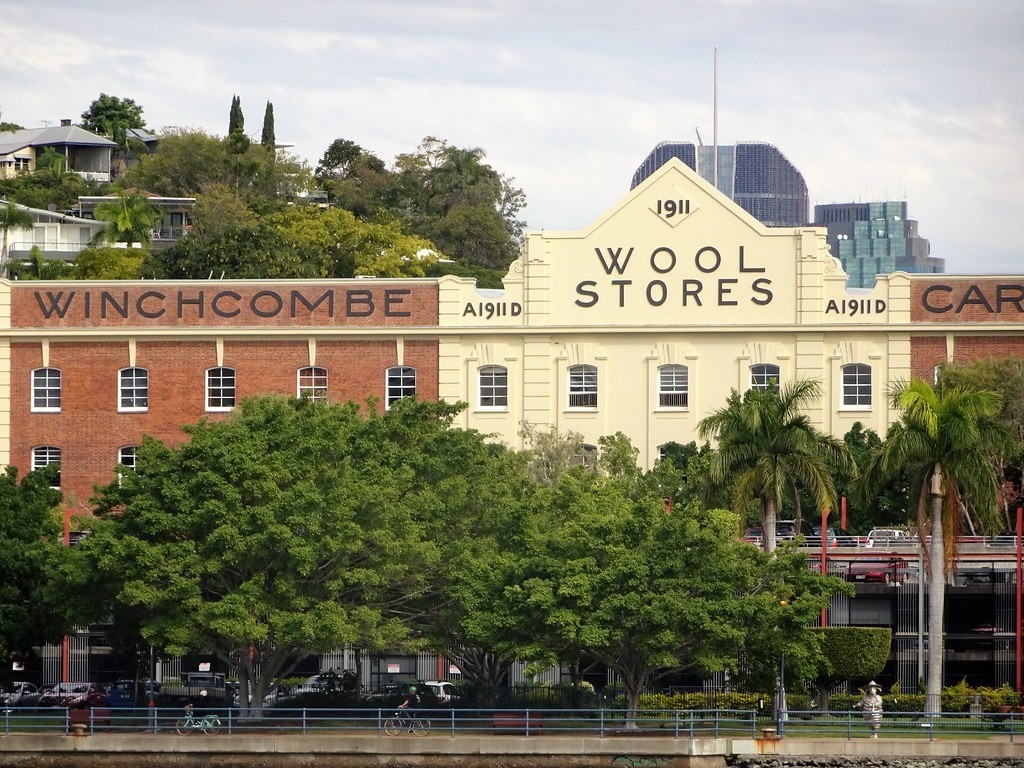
[188,673,225,698]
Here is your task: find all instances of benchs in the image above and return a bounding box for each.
[62,710,114,732]
[992,705,1024,728]
[491,712,543,736]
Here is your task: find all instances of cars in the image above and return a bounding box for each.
[426,680,461,704]
[865,530,909,547]
[233,683,288,707]
[846,551,909,583]
[304,675,356,696]
[366,686,408,705]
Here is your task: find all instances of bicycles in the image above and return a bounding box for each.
[176,711,221,736]
[384,706,431,737]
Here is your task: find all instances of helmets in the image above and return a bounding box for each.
[410,687,417,693]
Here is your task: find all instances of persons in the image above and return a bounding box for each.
[853,680,884,738]
[399,686,421,733]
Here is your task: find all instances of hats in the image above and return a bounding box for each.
[200,690,208,696]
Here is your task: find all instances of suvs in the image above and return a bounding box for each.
[0,682,42,713]
[107,680,148,712]
[776,519,818,547]
[39,682,107,712]
[812,528,853,547]
[741,528,764,547]
